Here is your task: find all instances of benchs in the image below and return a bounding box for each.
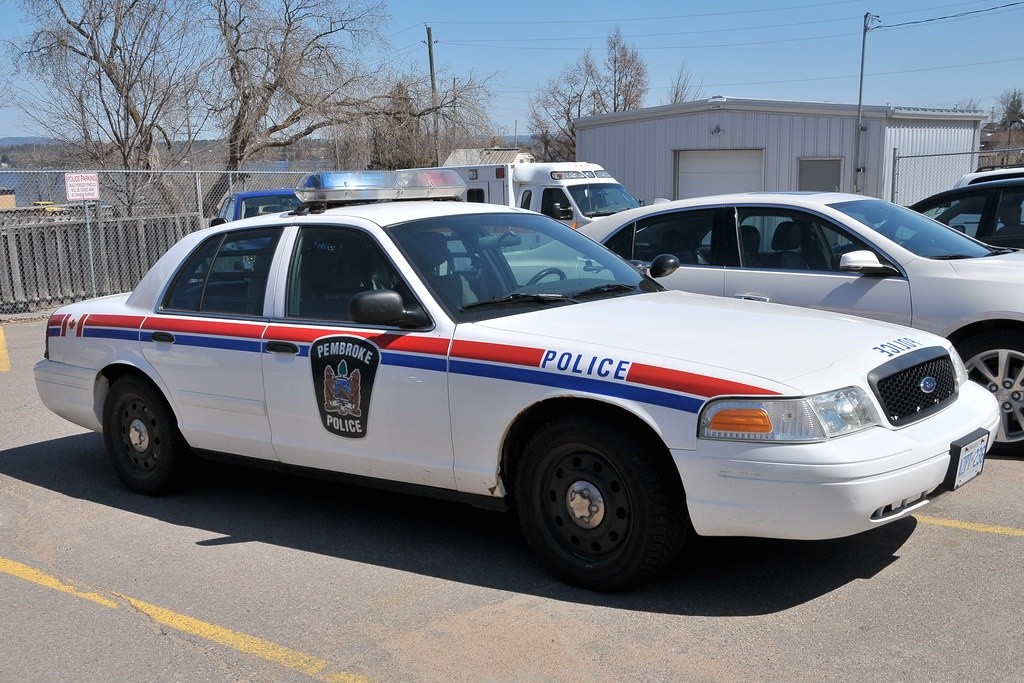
[658,246,711,265]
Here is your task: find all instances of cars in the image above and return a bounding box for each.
[209,190,303,273]
[440,192,1024,459]
[35,169,1000,595]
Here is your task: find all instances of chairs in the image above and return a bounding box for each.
[738,226,766,267]
[995,206,1024,234]
[414,231,481,311]
[768,222,813,272]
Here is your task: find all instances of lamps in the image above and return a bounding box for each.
[710,126,722,135]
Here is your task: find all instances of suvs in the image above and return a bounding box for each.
[831,163,1024,258]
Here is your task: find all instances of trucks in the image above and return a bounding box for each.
[432,161,644,252]
